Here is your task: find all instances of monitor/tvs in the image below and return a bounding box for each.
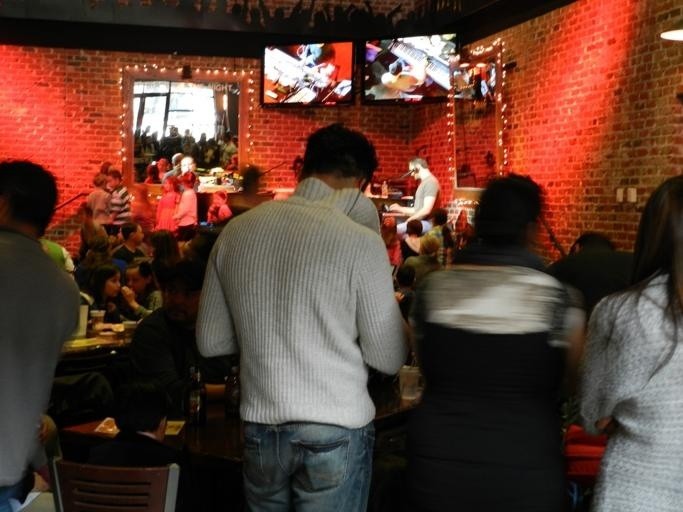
[258,38,358,107]
[361,33,461,107]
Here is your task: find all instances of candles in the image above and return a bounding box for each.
[627,188,637,203]
[617,188,626,202]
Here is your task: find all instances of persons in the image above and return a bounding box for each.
[408,235,442,286]
[174,172,199,268]
[461,232,473,251]
[580,175,683,512]
[396,288,416,318]
[152,174,182,232]
[76,237,129,286]
[91,382,173,467]
[32,236,79,484]
[403,221,424,257]
[179,158,200,189]
[546,232,634,316]
[197,122,410,512]
[414,175,585,512]
[130,269,203,378]
[209,191,233,223]
[158,158,172,182]
[382,218,402,268]
[146,162,161,183]
[233,168,277,210]
[427,209,451,260]
[79,204,108,254]
[3,160,80,509]
[396,264,419,295]
[151,229,182,278]
[120,260,164,318]
[107,170,131,236]
[389,158,441,233]
[101,161,117,176]
[80,266,123,324]
[163,154,185,182]
[177,229,221,274]
[114,223,148,259]
[88,174,112,235]
[134,125,239,165]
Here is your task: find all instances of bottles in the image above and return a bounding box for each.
[382,181,387,198]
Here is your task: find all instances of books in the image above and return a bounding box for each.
[95,417,186,436]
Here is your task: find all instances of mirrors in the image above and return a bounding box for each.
[446,38,507,191]
[119,66,253,198]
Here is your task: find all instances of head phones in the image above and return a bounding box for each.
[410,160,421,180]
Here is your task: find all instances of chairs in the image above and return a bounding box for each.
[52,457,179,511]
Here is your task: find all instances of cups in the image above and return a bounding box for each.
[91,310,106,330]
[73,305,88,337]
[125,321,137,343]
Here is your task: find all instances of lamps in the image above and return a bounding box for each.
[660,18,683,41]
